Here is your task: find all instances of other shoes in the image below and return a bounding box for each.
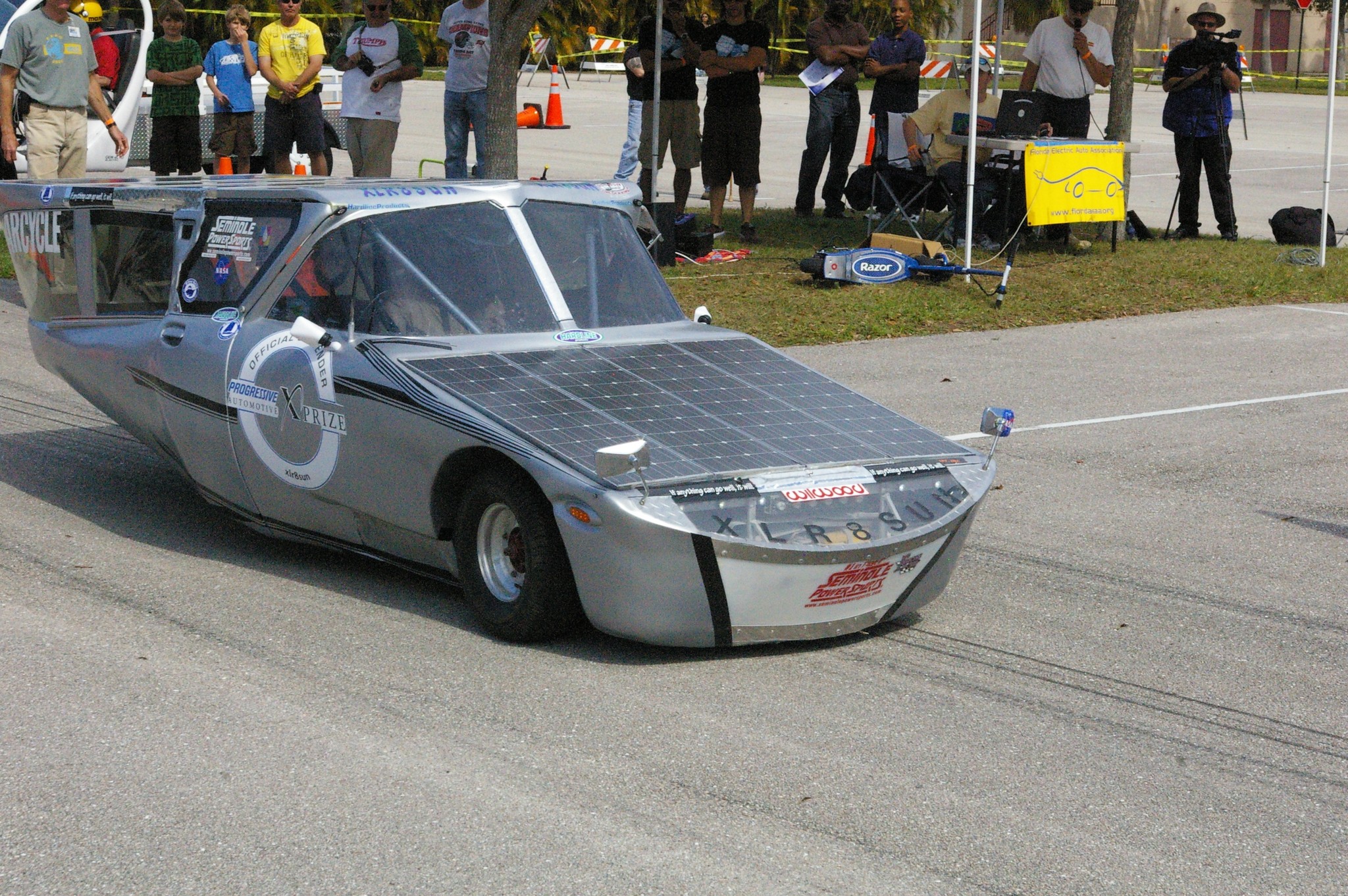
[903,213,920,224]
[944,227,978,249]
[1163,224,1199,241]
[703,224,726,240]
[741,223,759,242]
[863,214,883,221]
[1222,230,1237,242]
[797,210,812,220]
[970,232,1001,251]
[1044,233,1092,250]
[823,208,855,221]
[702,191,710,200]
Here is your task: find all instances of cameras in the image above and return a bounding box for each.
[357,55,374,76]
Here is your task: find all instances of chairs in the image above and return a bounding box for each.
[866,103,961,242]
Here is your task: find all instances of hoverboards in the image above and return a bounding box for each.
[799,238,1019,308]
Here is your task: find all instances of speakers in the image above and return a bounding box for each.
[639,201,678,266]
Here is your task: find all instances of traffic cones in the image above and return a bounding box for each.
[863,113,876,165]
[542,65,571,129]
[280,165,326,296]
[206,157,245,273]
[517,103,544,130]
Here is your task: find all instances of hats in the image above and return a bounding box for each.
[964,55,993,73]
[1186,1,1226,28]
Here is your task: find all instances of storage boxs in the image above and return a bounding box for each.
[870,233,950,263]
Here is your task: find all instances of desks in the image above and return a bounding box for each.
[944,134,1142,259]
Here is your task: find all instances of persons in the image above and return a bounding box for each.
[204,4,258,174]
[258,0,328,177]
[438,0,490,178]
[1162,2,1242,242]
[330,0,424,178]
[145,0,204,177]
[1020,0,1115,253]
[613,0,770,239]
[793,0,872,218]
[902,55,1053,255]
[69,0,121,117]
[864,0,926,223]
[0,0,129,180]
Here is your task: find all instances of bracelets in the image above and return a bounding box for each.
[1082,50,1092,60]
[681,57,686,66]
[908,144,917,152]
[104,118,117,129]
[387,72,395,81]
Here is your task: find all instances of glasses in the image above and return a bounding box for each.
[282,0,302,4]
[365,3,389,11]
[1070,6,1091,15]
[966,57,991,70]
[1196,21,1219,28]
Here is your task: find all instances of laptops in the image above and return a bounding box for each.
[979,91,1043,138]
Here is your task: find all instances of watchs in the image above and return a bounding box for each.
[1220,64,1228,71]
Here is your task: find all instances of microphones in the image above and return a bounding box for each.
[1075,19,1080,56]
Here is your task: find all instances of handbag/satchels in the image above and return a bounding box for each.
[1270,205,1338,246]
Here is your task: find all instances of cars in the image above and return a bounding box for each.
[0,172,999,649]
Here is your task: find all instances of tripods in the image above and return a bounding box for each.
[1162,61,1238,241]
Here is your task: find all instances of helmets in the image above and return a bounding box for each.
[70,0,102,22]
